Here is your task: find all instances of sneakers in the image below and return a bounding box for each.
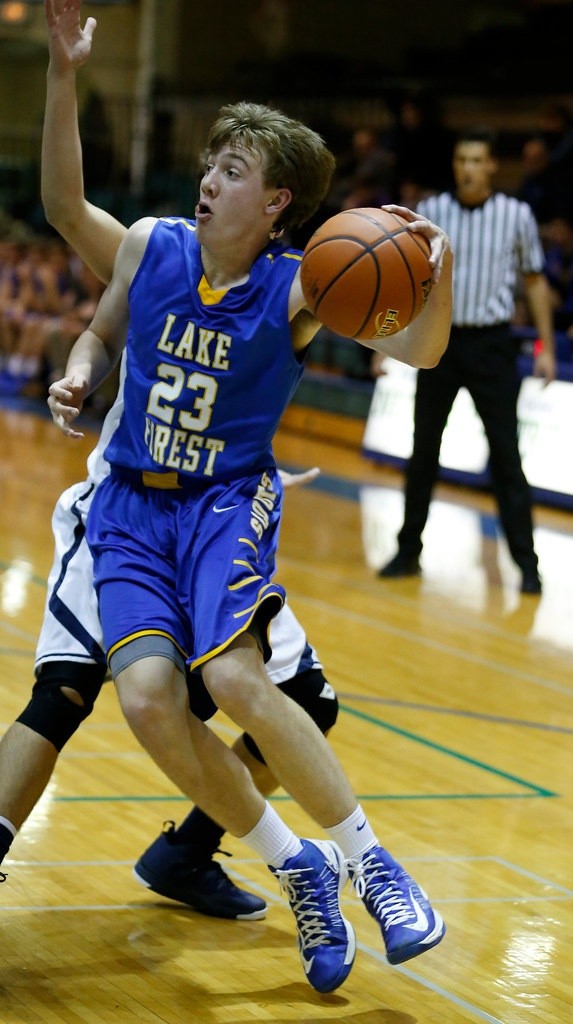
[343,847,445,964]
[133,820,267,920]
[268,837,357,993]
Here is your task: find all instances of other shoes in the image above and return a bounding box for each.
[378,550,421,579]
[520,569,542,593]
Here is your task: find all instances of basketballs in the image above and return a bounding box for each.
[299,207,437,341]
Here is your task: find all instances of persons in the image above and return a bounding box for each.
[1,0,338,924]
[43,98,452,993]
[376,124,554,595]
[321,103,572,255]
[0,238,121,411]
